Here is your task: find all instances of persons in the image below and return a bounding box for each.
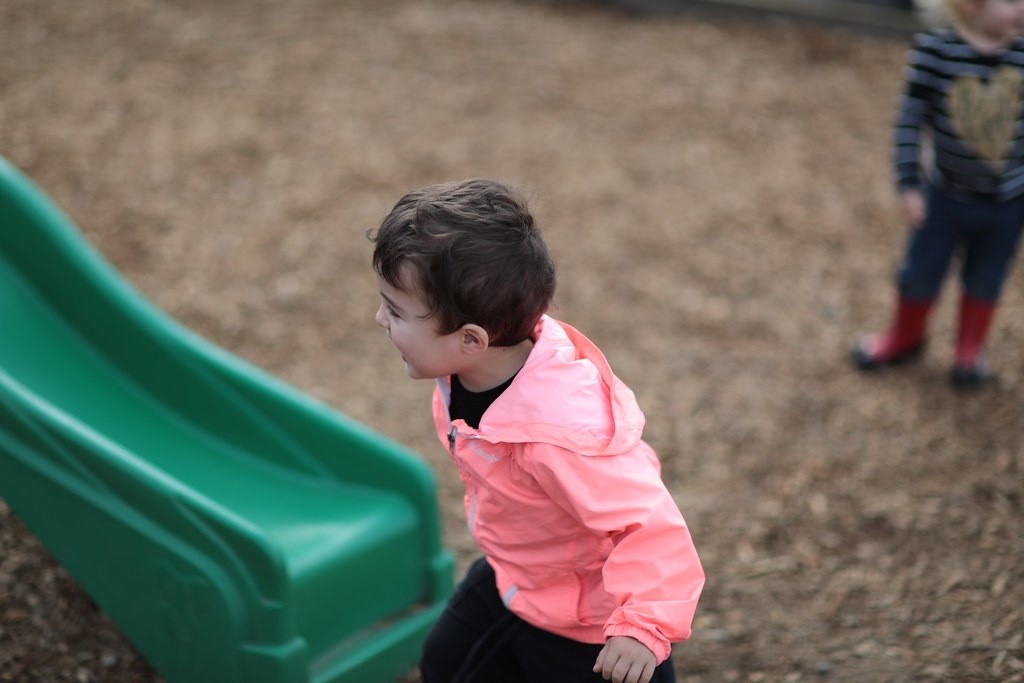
[846,0,1024,394]
[363,176,708,683]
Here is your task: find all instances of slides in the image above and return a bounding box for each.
[1,204,428,663]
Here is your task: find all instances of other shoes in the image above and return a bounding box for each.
[854,337,982,387]
